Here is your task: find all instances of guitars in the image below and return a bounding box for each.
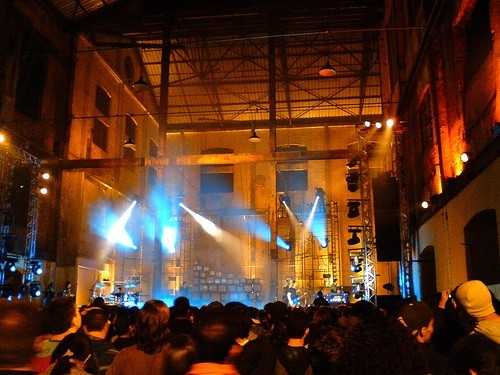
[287,292,307,309]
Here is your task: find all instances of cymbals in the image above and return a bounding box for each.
[87,288,98,292]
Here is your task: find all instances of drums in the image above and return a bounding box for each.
[90,297,98,304]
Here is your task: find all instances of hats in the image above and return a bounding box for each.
[456,280,495,317]
[402,301,439,328]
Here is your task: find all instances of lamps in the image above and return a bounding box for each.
[422,201,430,208]
[247,132,262,144]
[126,194,183,253]
[364,114,395,129]
[0,170,49,301]
[279,186,328,252]
[460,151,473,165]
[318,61,337,77]
[345,156,367,299]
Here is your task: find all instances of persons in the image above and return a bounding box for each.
[0,279,500,375]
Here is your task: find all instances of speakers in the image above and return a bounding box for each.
[6,166,31,256]
[371,179,401,262]
